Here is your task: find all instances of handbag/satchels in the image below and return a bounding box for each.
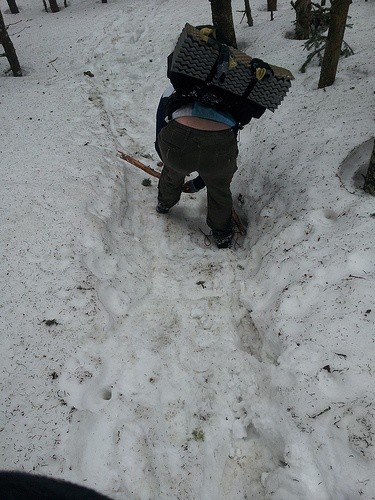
[167,22,295,126]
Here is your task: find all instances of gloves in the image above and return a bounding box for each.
[182,180,199,193]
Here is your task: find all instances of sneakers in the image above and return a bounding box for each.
[211,230,234,249]
[156,202,169,213]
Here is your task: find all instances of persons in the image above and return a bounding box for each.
[155,26,239,249]
